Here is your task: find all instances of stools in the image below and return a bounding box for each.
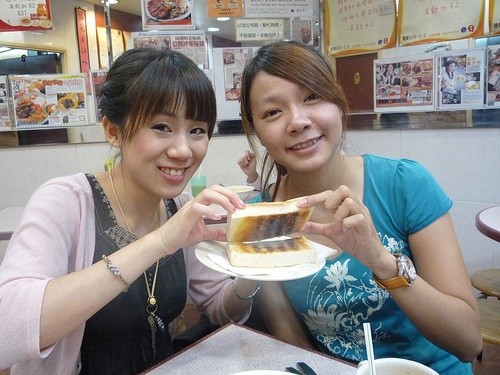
[470,268,500,361]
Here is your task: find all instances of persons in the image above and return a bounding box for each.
[237,148,278,191]
[442,60,457,90]
[0,47,259,375]
[241,41,483,375]
[382,64,396,85]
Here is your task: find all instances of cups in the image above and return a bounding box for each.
[357,358,439,375]
[190,177,205,198]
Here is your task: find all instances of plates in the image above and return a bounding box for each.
[226,90,241,99]
[145,0,191,21]
[194,240,327,281]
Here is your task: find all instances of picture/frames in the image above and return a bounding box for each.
[0,41,73,144]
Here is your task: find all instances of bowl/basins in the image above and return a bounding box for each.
[225,186,255,201]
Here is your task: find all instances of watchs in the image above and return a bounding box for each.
[372,253,416,290]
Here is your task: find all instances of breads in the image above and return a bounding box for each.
[226,236,317,268]
[225,196,315,242]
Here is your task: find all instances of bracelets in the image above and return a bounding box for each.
[233,279,261,300]
[101,255,129,288]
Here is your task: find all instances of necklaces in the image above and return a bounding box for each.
[109,172,160,315]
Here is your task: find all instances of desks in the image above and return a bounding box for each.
[0,202,228,240]
[475,205,500,243]
[137,322,358,375]
